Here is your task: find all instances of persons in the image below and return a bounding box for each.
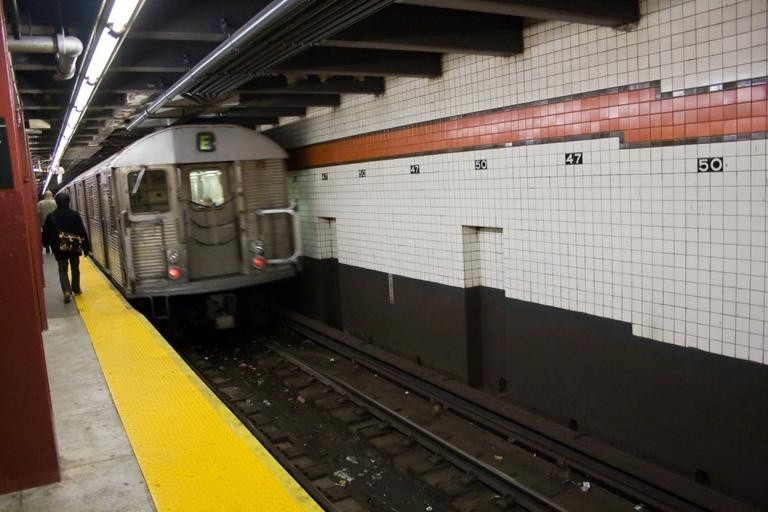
[36,190,58,255]
[40,192,91,304]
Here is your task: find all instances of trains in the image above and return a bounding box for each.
[54,124,305,346]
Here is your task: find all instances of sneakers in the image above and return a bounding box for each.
[64,292,70,303]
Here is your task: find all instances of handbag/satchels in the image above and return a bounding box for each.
[58,231,84,256]
